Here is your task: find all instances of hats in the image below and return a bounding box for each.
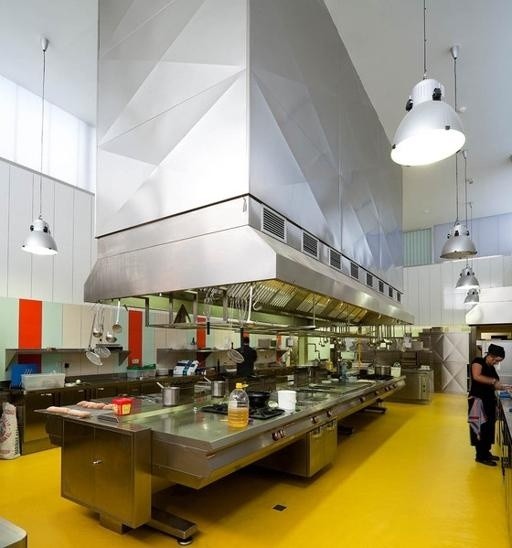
[488,344,505,359]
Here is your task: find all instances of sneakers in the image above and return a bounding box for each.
[475,452,499,466]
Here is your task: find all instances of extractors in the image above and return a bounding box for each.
[83,200,415,335]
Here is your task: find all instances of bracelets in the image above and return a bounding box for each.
[491,378,497,385]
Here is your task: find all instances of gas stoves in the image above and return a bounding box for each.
[362,373,393,381]
[202,400,284,420]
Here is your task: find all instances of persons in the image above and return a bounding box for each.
[235,336,258,377]
[466,343,505,466]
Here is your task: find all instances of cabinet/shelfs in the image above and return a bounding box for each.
[10,363,293,455]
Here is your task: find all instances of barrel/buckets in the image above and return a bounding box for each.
[390,366,401,377]
[277,390,297,414]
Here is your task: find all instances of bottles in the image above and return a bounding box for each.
[327,362,333,370]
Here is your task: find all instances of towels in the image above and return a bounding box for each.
[465,397,490,442]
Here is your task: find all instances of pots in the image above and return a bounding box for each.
[375,366,390,376]
[211,380,226,397]
[411,341,423,350]
[244,391,270,406]
[161,387,181,406]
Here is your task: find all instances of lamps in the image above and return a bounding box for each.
[19,37,60,258]
[388,0,469,168]
[437,37,487,308]
